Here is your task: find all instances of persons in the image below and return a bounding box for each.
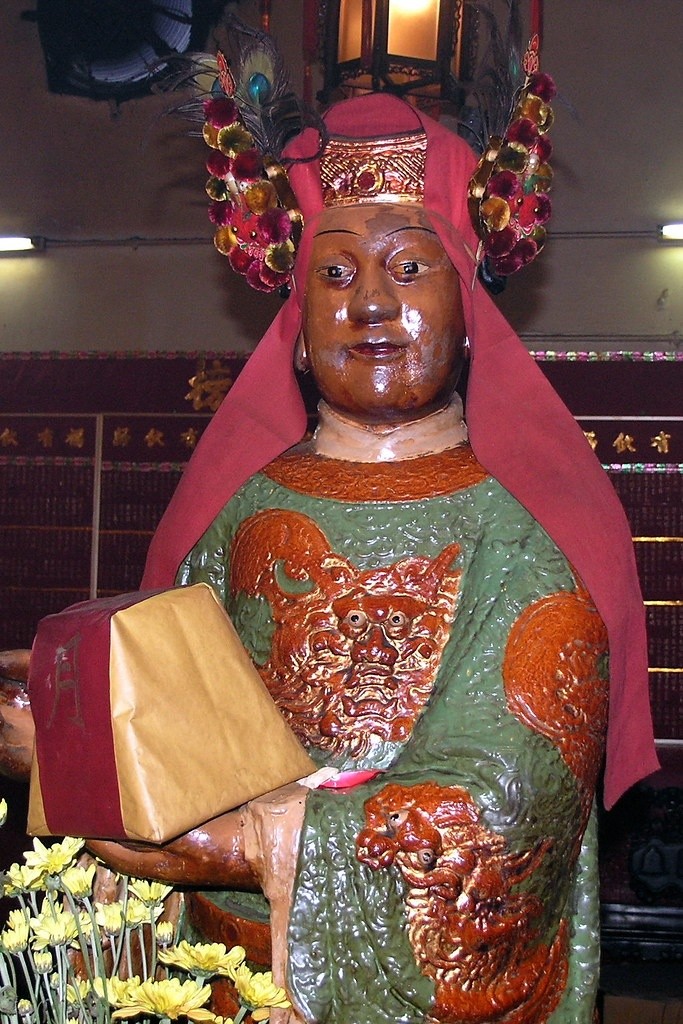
[0,54,659,1024]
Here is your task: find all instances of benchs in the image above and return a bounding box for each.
[596,778,683,1003]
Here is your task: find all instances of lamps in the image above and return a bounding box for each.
[257,1,524,162]
[0,236,45,251]
[657,223,683,241]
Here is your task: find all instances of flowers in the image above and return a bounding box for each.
[0,797,292,1024]
